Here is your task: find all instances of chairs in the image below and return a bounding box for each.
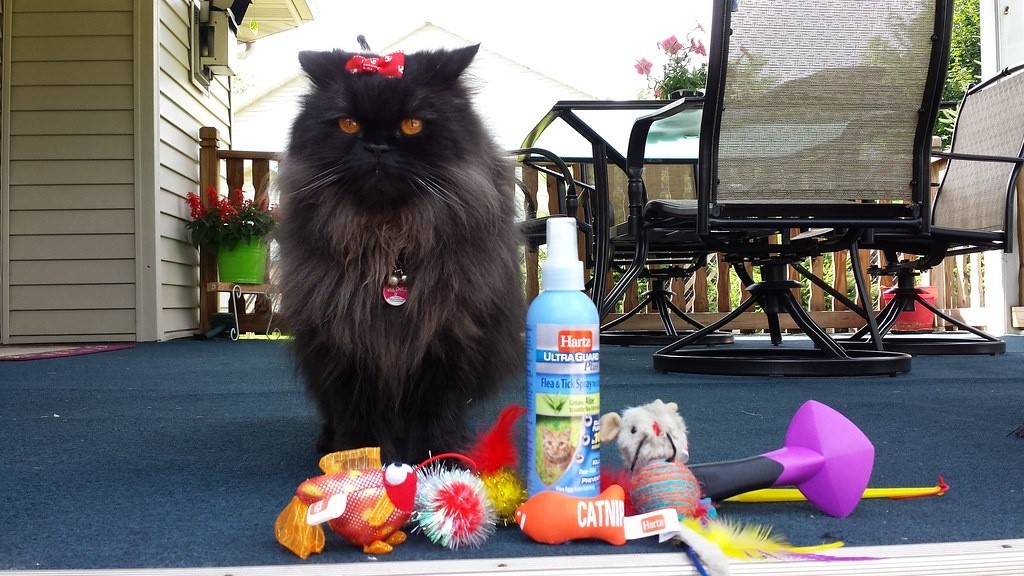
[812,62,1024,355]
[626,0,955,377]
[579,159,736,345]
[499,148,593,270]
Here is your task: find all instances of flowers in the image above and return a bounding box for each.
[184,185,282,250]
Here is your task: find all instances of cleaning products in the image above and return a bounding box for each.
[522,218,605,501]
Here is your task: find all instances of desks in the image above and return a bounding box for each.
[518,98,888,322]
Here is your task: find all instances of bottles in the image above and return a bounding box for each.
[524,217,602,499]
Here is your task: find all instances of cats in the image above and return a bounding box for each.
[253,41,534,475]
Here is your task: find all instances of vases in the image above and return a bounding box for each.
[214,234,269,285]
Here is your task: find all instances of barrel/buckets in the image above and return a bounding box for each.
[880,286,939,330]
